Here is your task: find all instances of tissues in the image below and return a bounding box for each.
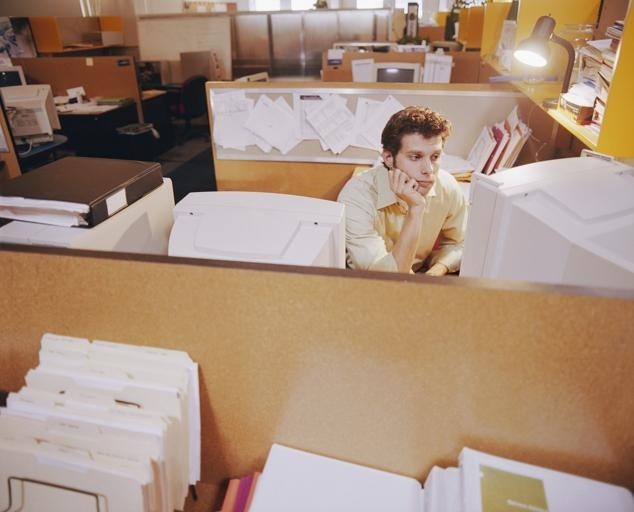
[557,81,596,126]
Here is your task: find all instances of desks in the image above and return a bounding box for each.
[0,97,68,178]
[10,56,168,132]
[27,15,138,57]
[52,96,135,157]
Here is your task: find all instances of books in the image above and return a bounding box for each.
[1,333,201,511]
[221,443,632,510]
[579,19,625,135]
[467,105,531,175]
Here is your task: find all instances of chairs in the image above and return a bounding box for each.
[162,75,220,128]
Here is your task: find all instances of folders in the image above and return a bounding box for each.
[1,155,160,229]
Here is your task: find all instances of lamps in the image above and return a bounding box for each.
[513,15,577,110]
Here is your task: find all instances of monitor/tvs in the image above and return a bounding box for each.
[458,149,633,289]
[2,176,177,254]
[0,66,27,87]
[0,85,62,147]
[429,41,462,53]
[235,72,270,83]
[371,62,423,82]
[166,191,348,268]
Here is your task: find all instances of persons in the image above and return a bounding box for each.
[336,107,468,280]
